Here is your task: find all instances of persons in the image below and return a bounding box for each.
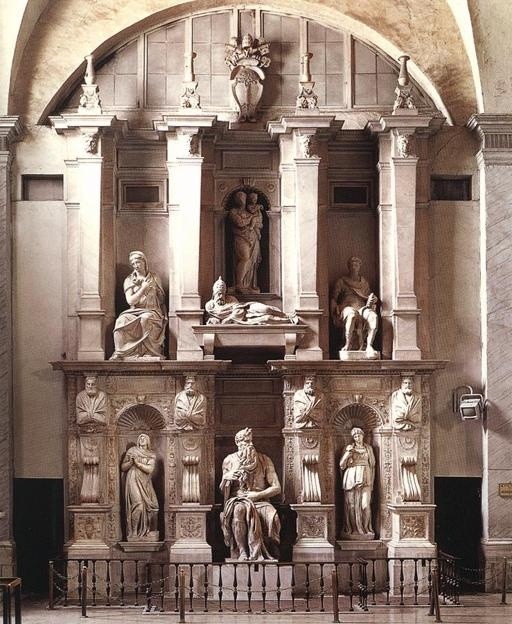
[72,371,209,546]
[218,429,283,561]
[229,191,265,295]
[200,278,306,327]
[287,373,422,540]
[110,250,168,362]
[331,256,382,352]
